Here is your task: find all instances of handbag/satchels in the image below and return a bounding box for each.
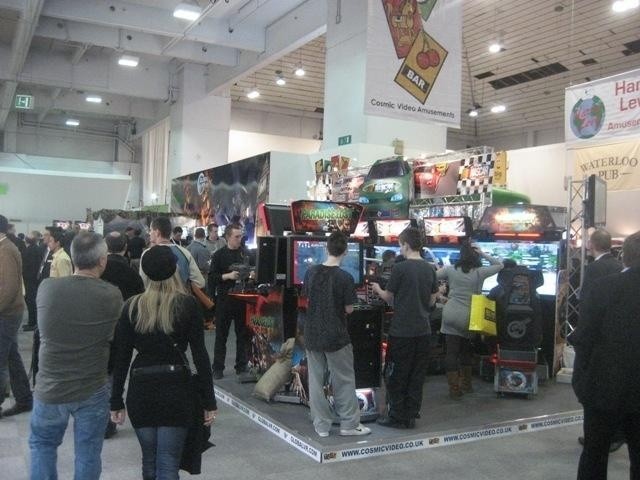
[468,293,498,336]
[324,384,379,424]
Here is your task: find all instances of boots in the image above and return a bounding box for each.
[458,375,473,393]
[448,371,463,399]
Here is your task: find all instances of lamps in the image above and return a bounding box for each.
[295,46,304,77]
[491,67,505,113]
[276,58,286,85]
[66,118,80,125]
[468,80,478,117]
[247,72,260,99]
[86,97,102,103]
[118,55,139,67]
[489,7,500,53]
[173,3,201,21]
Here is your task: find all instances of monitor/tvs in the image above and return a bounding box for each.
[588,174,609,227]
[255,234,284,286]
[373,243,406,277]
[469,241,559,301]
[266,204,295,232]
[286,237,362,300]
[424,245,464,274]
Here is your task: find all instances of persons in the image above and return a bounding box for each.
[436,244,505,401]
[572,232,640,479]
[0,215,256,480]
[370,228,439,429]
[579,231,625,452]
[374,250,394,277]
[488,259,515,303]
[304,231,372,436]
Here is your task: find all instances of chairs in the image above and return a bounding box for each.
[480,265,549,399]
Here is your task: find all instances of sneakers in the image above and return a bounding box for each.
[339,424,372,436]
[405,418,415,428]
[214,370,224,380]
[377,416,409,429]
[315,427,329,438]
[2,401,33,416]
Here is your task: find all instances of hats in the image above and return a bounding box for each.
[142,245,178,281]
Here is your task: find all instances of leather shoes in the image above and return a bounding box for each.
[578,437,585,444]
[609,440,625,452]
